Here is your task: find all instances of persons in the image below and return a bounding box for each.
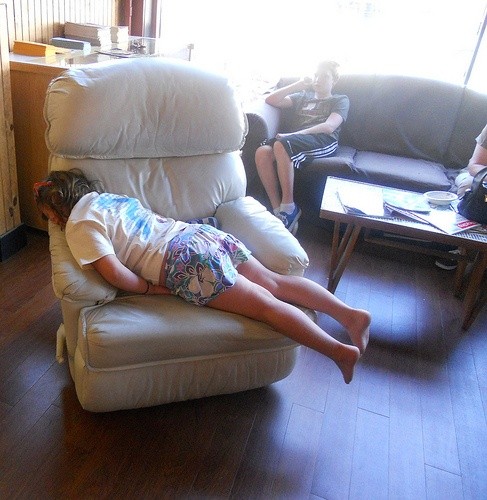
[254,61,352,236]
[34,170,374,384]
[434,124,487,271]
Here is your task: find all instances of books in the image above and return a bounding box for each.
[336,188,486,237]
[11,20,130,58]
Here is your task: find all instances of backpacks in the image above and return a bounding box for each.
[457,167,487,225]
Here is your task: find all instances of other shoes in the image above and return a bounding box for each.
[436,248,463,269]
[277,203,302,233]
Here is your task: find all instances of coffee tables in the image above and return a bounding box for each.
[320,176,487,330]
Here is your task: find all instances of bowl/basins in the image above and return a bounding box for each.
[423,190,458,206]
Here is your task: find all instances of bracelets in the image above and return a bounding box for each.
[142,280,149,295]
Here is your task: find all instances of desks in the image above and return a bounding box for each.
[9,52,174,235]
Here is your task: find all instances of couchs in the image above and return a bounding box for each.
[41,64,317,414]
[244,74,487,213]
